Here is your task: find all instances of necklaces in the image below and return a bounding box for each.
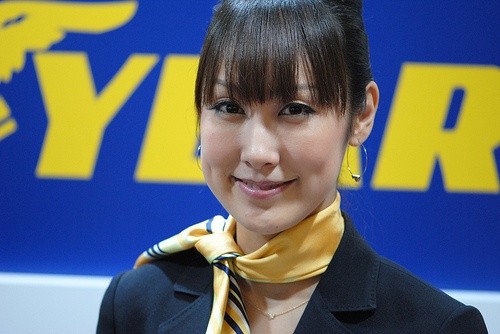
[242,295,311,319]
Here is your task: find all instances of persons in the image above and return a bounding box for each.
[94,1,488,333]
